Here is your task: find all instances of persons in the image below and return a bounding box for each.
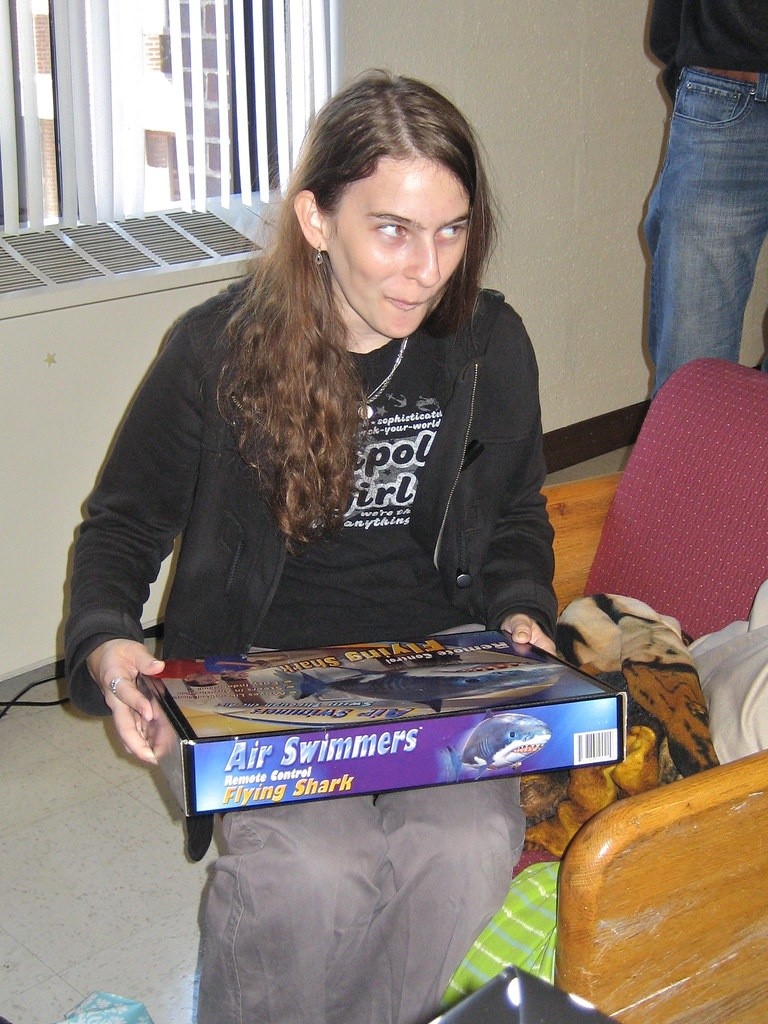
[58,68,560,1024]
[642,0,768,399]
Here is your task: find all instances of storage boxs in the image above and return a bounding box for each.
[133,630,628,817]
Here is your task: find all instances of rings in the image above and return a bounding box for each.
[109,677,125,696]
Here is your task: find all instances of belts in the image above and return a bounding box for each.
[688,64,760,85]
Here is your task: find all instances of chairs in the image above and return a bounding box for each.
[510,357,768,1024]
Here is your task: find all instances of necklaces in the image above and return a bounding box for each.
[355,336,409,421]
